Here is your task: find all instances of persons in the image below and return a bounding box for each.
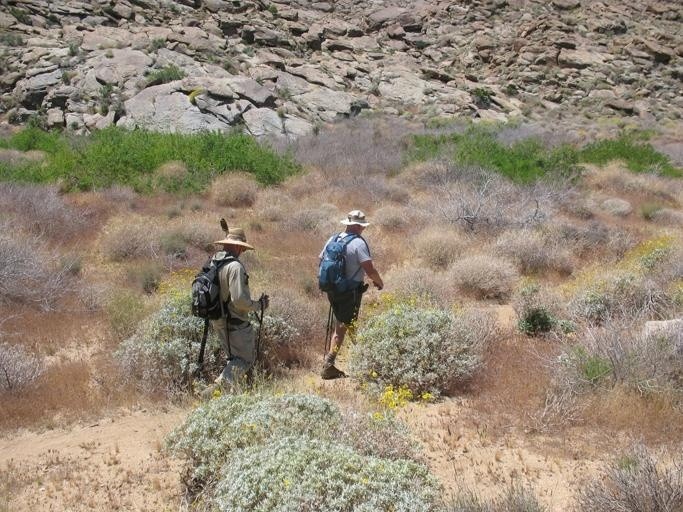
[197,226,269,403]
[315,210,384,381]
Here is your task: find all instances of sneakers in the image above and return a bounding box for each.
[321,363,350,380]
[196,384,219,402]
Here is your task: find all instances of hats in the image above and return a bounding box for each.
[337,209,373,226]
[212,226,254,251]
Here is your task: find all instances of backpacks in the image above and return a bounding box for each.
[189,255,242,321]
[317,230,370,295]
